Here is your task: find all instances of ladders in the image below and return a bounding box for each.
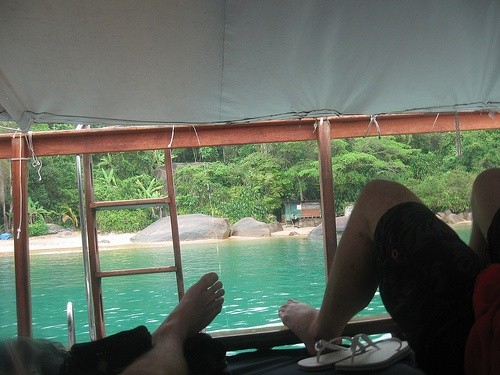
[84,123,184,339]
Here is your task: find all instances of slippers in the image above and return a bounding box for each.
[298,334,411,370]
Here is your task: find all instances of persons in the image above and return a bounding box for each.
[277,168,500,375]
[118,272,225,375]
[292,214,298,225]
